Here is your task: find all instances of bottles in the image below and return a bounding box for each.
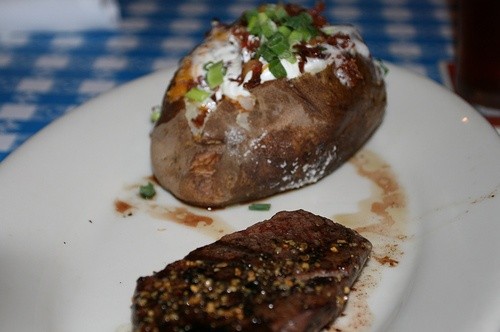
[446,0,500,108]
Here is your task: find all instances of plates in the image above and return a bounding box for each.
[1,62,500,332]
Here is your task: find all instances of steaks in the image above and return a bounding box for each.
[130,209,372,331]
